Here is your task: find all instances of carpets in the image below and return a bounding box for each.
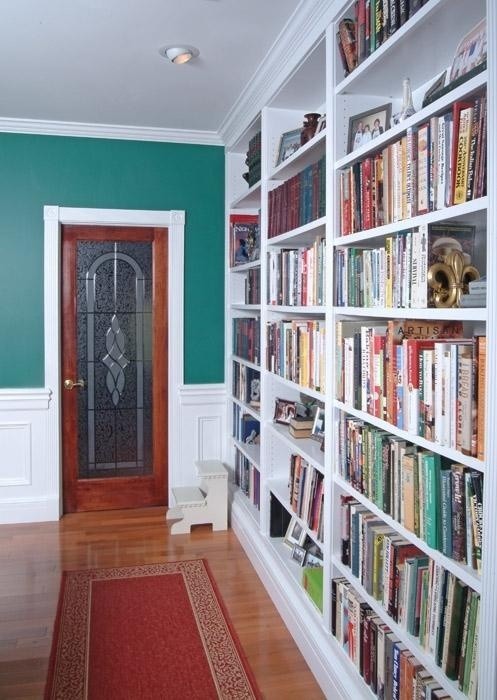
[45,558,262,699]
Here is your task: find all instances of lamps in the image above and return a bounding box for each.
[165,46,192,65]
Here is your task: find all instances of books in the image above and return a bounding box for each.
[335,0,430,77]
[235,101,488,700]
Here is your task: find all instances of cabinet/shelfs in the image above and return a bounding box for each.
[221,0,496,700]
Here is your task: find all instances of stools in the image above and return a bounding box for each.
[166,459,229,534]
